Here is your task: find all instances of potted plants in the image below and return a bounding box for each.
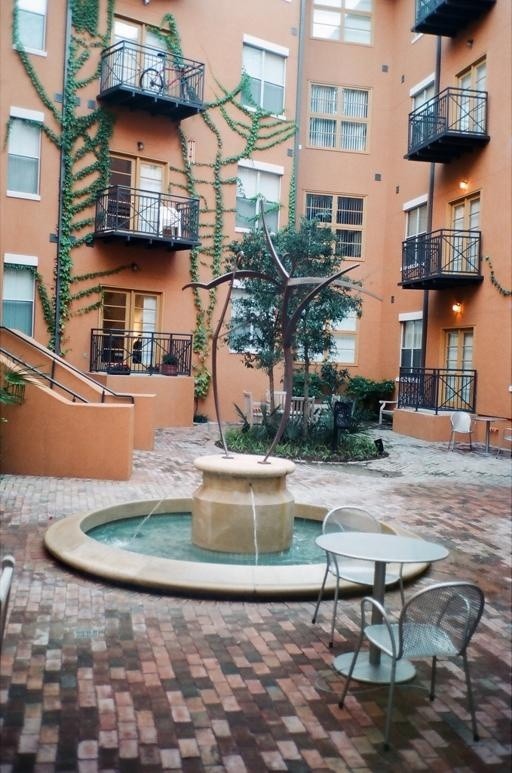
[162,353,179,375]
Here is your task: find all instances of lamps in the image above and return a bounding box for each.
[453,303,464,313]
[137,141,143,152]
[459,177,469,188]
[143,0,149,6]
[466,39,473,48]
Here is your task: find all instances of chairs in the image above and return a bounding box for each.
[0,555,14,653]
[496,427,512,458]
[337,581,483,750]
[158,207,182,239]
[449,412,474,452]
[245,389,341,432]
[378,395,409,425]
[312,505,408,649]
